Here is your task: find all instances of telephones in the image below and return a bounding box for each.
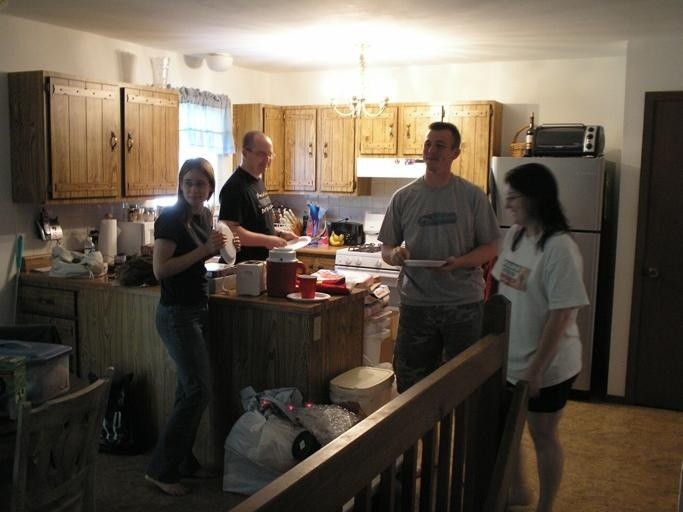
[35,207,64,241]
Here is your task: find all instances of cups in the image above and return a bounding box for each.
[299,275,316,298]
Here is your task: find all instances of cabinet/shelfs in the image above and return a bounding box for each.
[294,234,350,275]
[358,102,443,158]
[443,99,503,203]
[8,69,180,205]
[13,272,79,379]
[80,278,370,465]
[285,104,372,196]
[233,102,284,195]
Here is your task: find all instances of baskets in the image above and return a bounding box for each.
[511,125,529,156]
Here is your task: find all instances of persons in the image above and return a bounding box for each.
[217,129,300,269]
[490,163,591,511]
[376,121,504,478]
[141,158,240,493]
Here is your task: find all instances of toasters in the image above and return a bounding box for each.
[235,258,266,296]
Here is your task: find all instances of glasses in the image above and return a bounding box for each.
[246,147,276,160]
[182,181,208,189]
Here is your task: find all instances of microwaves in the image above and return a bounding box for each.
[533,122,605,158]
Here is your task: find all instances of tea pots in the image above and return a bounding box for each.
[266,250,306,297]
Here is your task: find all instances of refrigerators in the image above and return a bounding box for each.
[489,158,619,401]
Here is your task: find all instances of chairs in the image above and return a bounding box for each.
[10,366,117,512]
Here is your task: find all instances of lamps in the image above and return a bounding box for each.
[323,45,390,120]
[205,52,233,72]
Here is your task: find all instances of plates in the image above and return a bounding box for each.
[274,235,311,249]
[216,221,238,265]
[287,292,330,303]
[403,259,446,268]
[205,262,228,271]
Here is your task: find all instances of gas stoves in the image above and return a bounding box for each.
[335,243,405,271]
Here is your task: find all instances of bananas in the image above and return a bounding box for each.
[329,230,345,246]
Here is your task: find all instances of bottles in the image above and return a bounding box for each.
[525,111,536,156]
[84,237,95,254]
[303,209,309,236]
[128,207,134,222]
[137,208,144,223]
